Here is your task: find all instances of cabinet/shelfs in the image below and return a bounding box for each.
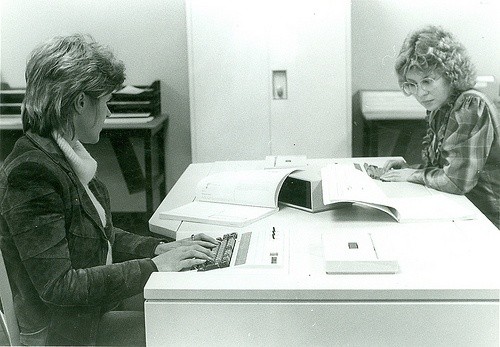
[185,0,353,159]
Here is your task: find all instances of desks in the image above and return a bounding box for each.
[359,91,436,157]
[1,114,168,220]
[143,155,500,346]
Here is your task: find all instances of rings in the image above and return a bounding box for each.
[191,234,194,241]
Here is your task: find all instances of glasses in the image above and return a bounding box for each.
[402,73,446,97]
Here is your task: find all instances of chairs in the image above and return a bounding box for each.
[0,217,29,346]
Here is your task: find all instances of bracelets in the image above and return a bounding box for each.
[159,241,165,244]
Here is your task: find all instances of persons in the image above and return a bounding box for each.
[0,31,217,346]
[380,25,500,229]
